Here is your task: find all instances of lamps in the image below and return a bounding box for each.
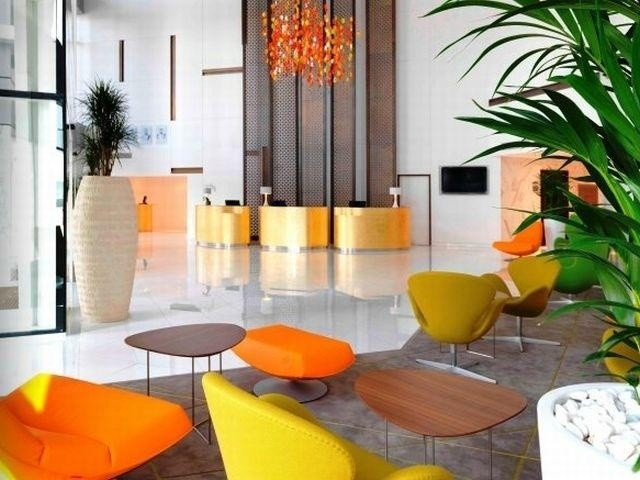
[202,188,211,205]
[258,1,361,88]
[389,187,402,207]
[260,186,273,206]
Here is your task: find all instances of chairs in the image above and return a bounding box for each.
[1,371,194,479]
[202,372,455,479]
[403,214,609,387]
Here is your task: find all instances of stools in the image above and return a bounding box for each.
[232,324,356,406]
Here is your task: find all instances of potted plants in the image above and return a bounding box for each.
[420,0,639,479]
[60,72,144,322]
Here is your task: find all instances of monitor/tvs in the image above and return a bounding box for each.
[348,200,366,207]
[271,200,286,206]
[225,199,240,205]
[441,166,488,193]
